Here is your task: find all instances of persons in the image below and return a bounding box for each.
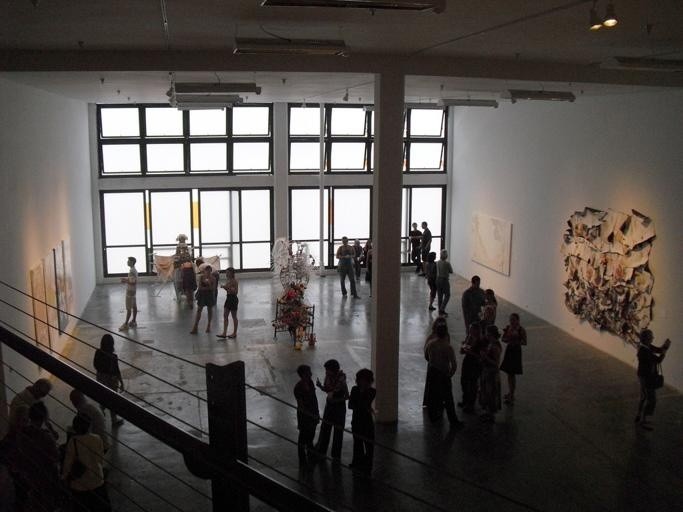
[0,378,111,512]
[118,257,138,331]
[92,333,125,427]
[634,329,672,424]
[179,255,239,338]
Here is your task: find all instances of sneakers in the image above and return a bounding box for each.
[428,306,436,310]
[226,334,237,338]
[127,320,137,328]
[117,322,130,332]
[215,333,226,338]
[438,310,448,316]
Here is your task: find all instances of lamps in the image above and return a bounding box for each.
[343,95,348,100]
[604,3,618,27]
[500,89,577,103]
[260,1,442,12]
[234,38,353,58]
[169,82,261,110]
[437,98,498,107]
[590,4,602,30]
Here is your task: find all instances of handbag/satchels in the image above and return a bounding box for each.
[69,437,89,481]
[645,357,665,389]
